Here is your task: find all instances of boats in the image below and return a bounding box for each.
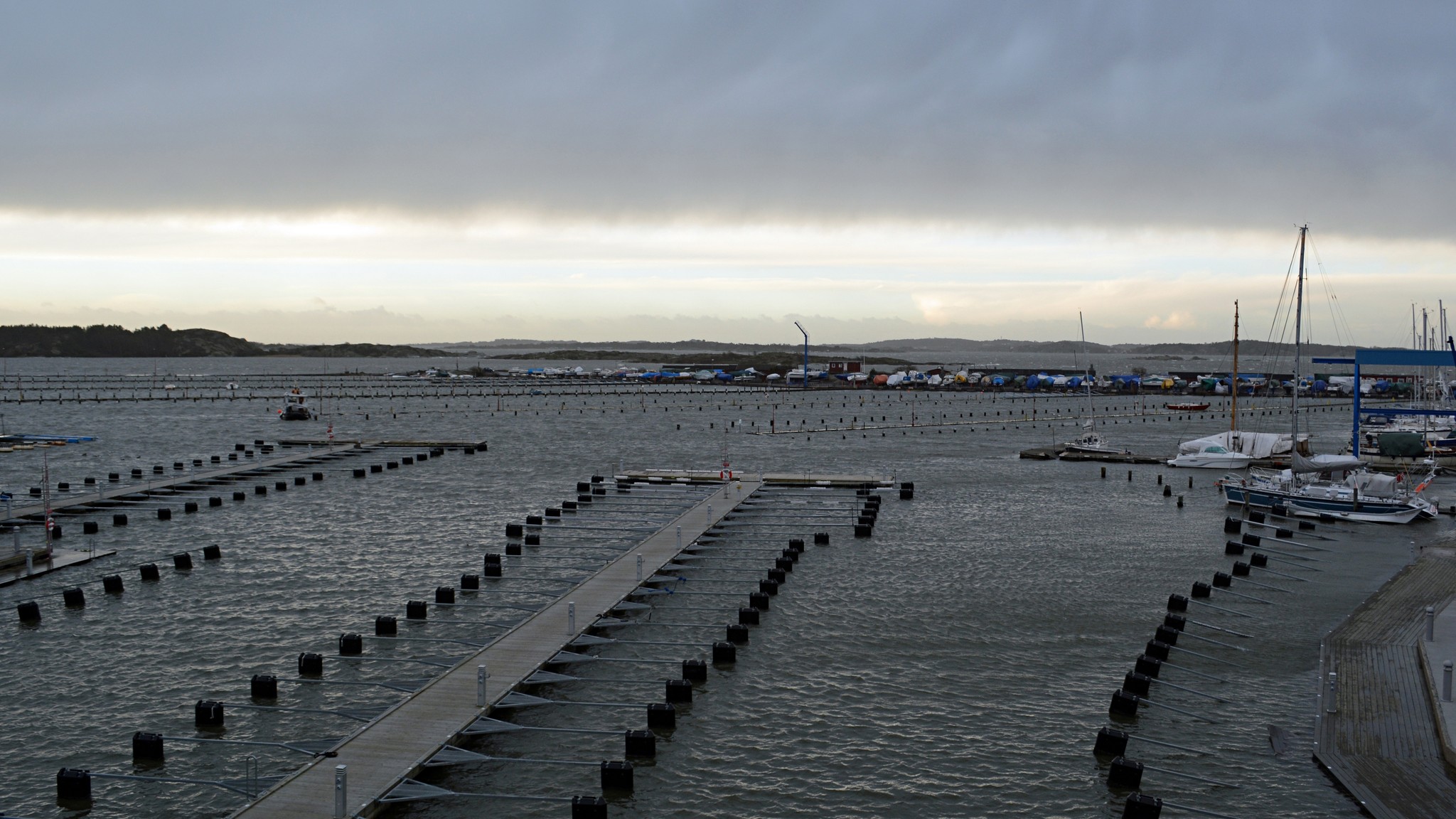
[1166,438,1255,469]
[1165,403,1210,411]
[226,383,238,390]
[277,379,313,421]
[165,384,176,390]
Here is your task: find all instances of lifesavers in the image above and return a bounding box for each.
[46,518,55,531]
[720,469,732,481]
[326,430,334,438]
[1385,423,1391,428]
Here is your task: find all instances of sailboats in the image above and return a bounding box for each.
[411,222,1456,527]
